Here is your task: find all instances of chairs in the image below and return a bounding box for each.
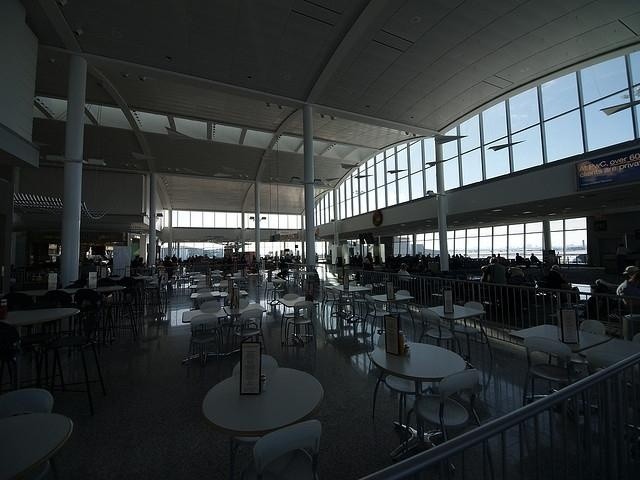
[181,271,322,362]
[248,420,324,478]
[2,273,177,412]
[508,310,639,478]
[322,274,495,415]
[231,354,279,376]
[413,367,490,473]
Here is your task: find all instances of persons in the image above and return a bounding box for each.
[616,266,640,314]
[616,243,632,279]
[131,253,300,275]
[350,251,571,290]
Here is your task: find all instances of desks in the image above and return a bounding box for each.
[369,342,467,473]
[202,367,325,478]
[4,414,75,479]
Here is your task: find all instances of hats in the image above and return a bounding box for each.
[623,266,638,275]
